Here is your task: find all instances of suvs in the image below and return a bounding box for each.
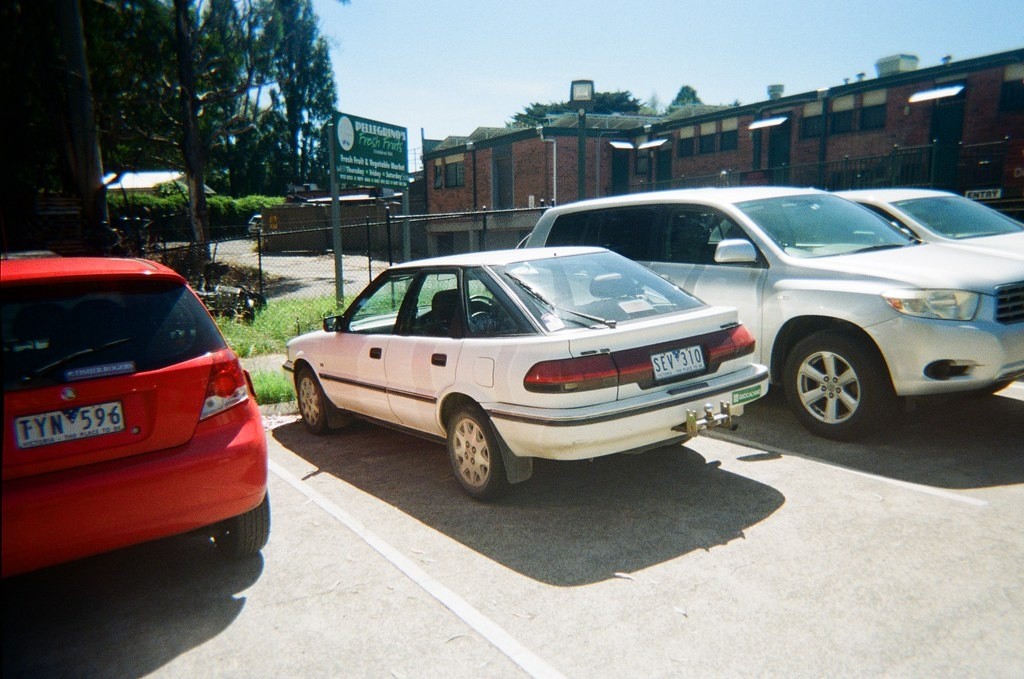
[510,188,1024,442]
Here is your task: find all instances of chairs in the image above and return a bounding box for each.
[582,273,634,321]
[423,289,462,336]
[671,217,713,265]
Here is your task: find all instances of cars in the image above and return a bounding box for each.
[785,185,1024,263]
[285,245,772,504]
[248,214,262,237]
[1,257,272,590]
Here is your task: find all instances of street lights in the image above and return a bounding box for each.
[570,79,595,203]
